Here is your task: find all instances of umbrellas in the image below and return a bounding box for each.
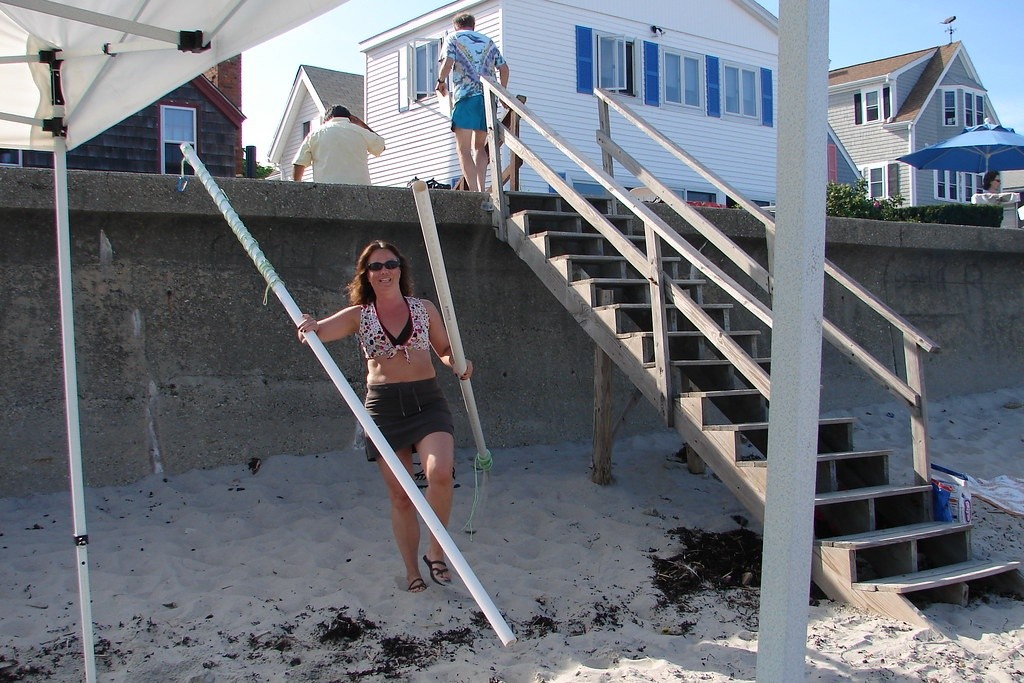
[895,118,1024,194]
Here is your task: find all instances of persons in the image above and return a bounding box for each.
[293,104,389,186]
[295,239,474,594]
[982,170,1001,194]
[436,14,510,193]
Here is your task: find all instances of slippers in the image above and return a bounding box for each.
[423,555,452,587]
[408,578,427,592]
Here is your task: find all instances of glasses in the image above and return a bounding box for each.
[366,259,401,270]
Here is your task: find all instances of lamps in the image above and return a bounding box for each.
[652,25,667,38]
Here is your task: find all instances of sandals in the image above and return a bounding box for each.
[426,178,452,189]
[407,177,419,188]
